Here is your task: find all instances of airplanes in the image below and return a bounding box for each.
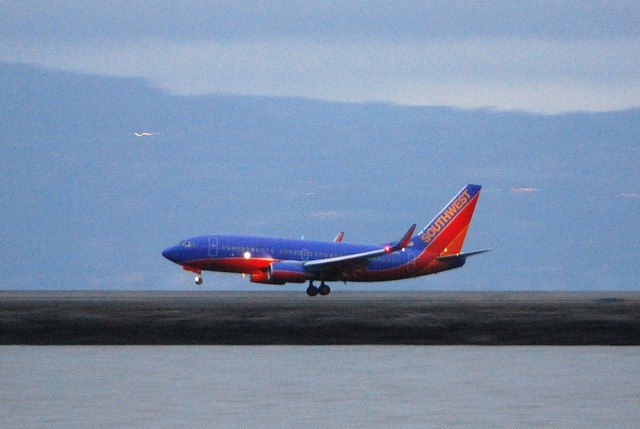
[161,183,493,297]
